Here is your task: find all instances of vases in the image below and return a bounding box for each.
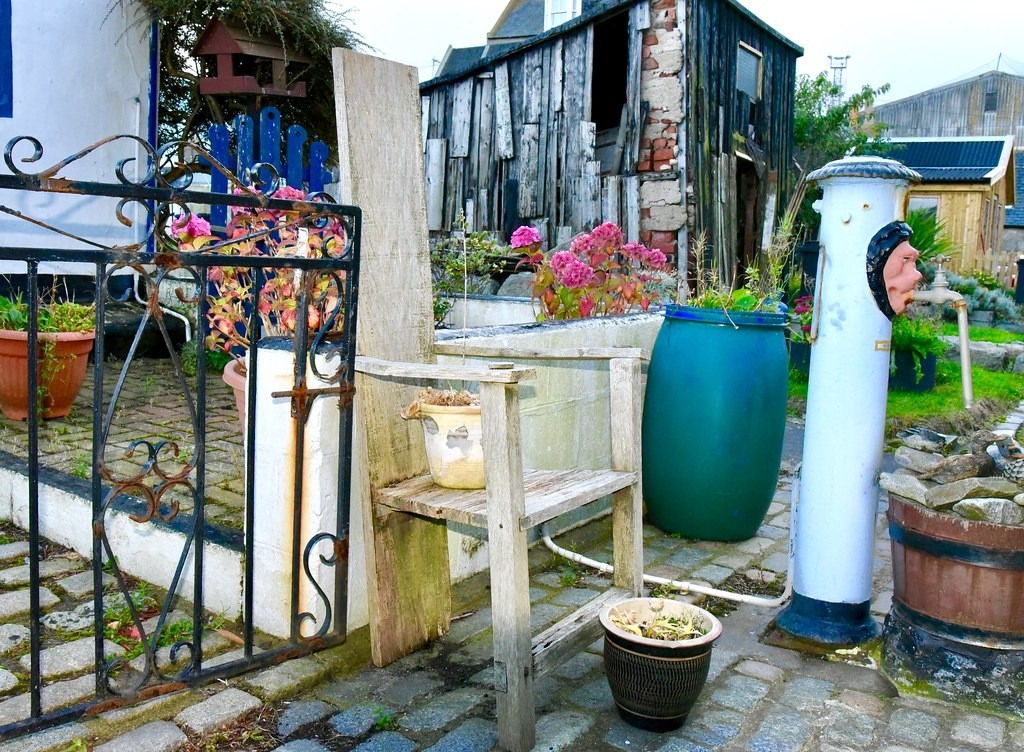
[222,356,247,435]
[884,492,1024,650]
[790,342,811,368]
[420,393,485,491]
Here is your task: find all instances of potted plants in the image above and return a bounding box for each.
[0,284,96,421]
[599,579,723,733]
[887,312,954,394]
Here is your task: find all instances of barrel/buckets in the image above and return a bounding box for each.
[642,305,789,542]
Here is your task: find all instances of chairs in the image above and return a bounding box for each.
[332,47,645,752]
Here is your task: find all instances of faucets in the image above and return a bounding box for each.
[913,254,968,310]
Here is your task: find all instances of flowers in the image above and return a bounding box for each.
[792,296,814,342]
[169,185,348,374]
[510,222,666,322]
